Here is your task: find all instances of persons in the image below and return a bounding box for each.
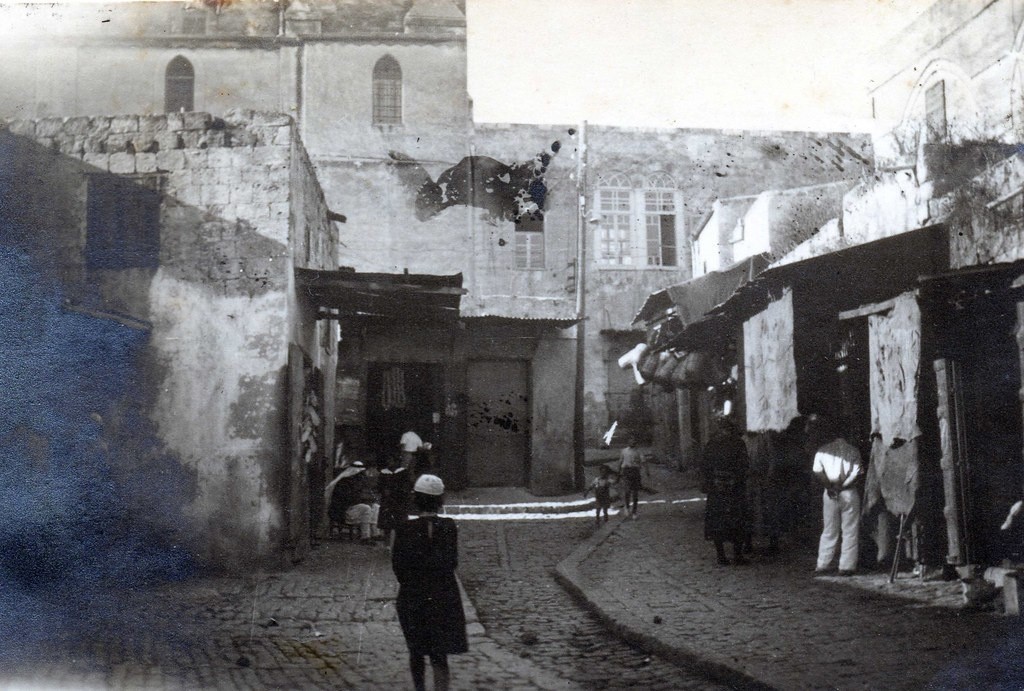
[399,425,424,483]
[392,475,468,691]
[696,411,867,576]
[376,468,408,533]
[326,461,385,547]
[618,434,651,520]
[584,465,620,525]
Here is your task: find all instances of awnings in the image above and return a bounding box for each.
[297,265,468,337]
[631,223,942,355]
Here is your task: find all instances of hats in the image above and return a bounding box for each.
[413,474,445,496]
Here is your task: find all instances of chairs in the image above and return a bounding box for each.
[326,505,361,540]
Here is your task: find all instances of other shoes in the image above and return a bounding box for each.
[717,555,731,565]
[625,505,629,517]
[632,513,638,520]
[741,543,754,553]
[735,554,750,565]
[768,538,780,549]
